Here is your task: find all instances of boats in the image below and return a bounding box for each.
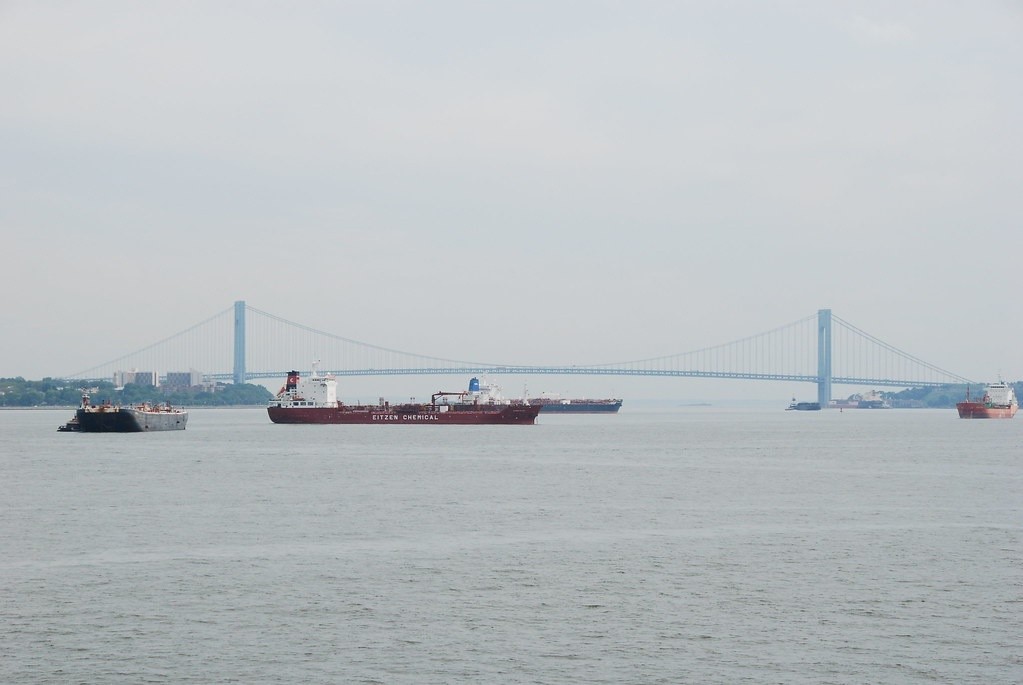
[56,394,190,436]
[795,401,822,411]
[421,378,622,414]
[266,358,543,426]
[955,379,1018,419]
